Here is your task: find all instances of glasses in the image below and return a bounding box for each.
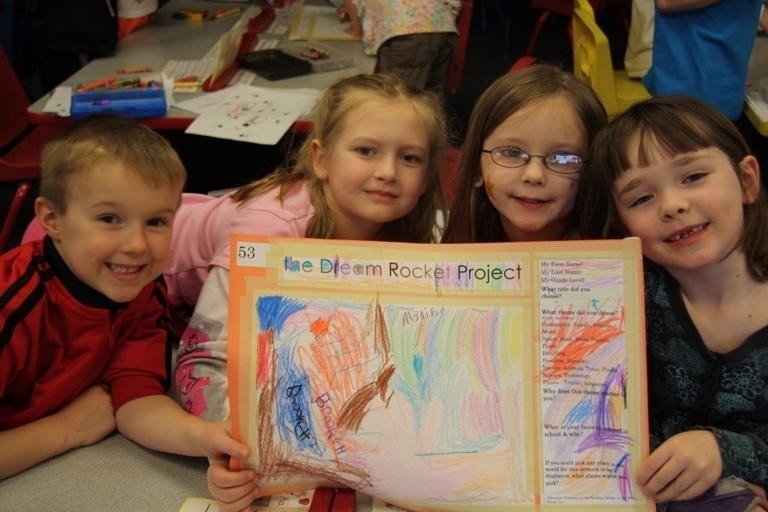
[481,145,587,174]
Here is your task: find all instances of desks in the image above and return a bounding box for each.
[25,1,380,134]
[0,432,373,512]
[737,34,768,137]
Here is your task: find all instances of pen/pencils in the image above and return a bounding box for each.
[300,44,331,60]
[209,7,240,22]
[73,67,203,93]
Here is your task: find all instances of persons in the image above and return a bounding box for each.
[575,97,768,510]
[438,66,619,242]
[0,119,190,482]
[23,76,452,509]
[1,1,768,150]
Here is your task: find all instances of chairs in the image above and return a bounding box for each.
[0,49,67,251]
